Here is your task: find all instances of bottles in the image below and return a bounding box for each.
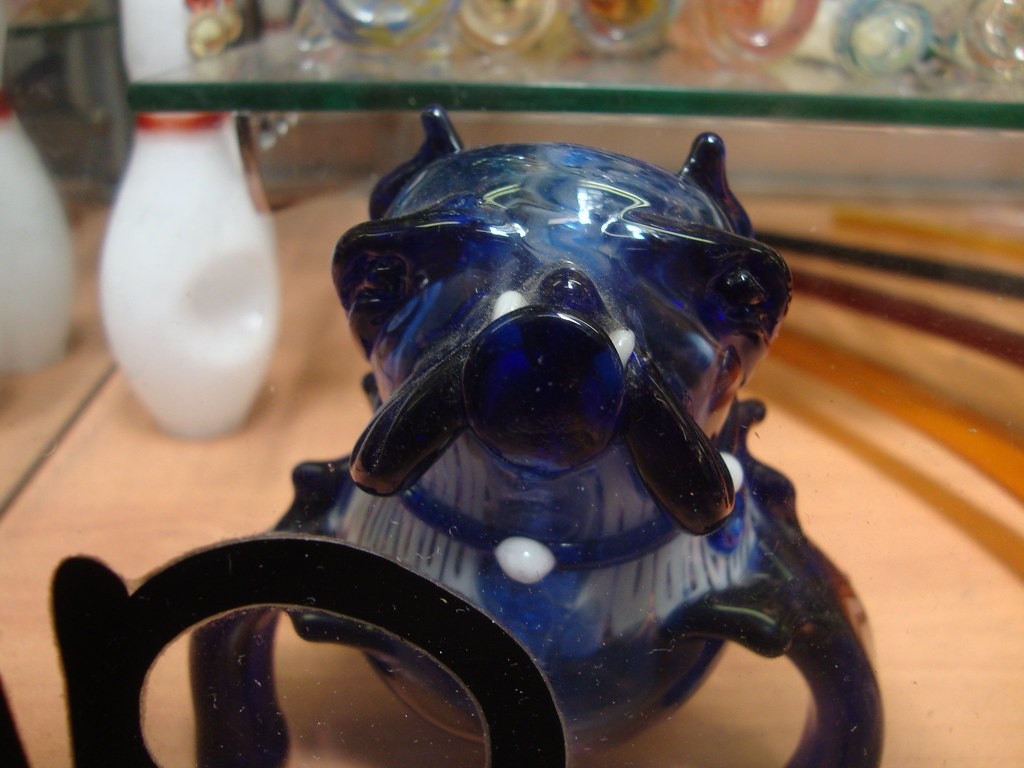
[0,97,78,376]
[97,111,282,440]
